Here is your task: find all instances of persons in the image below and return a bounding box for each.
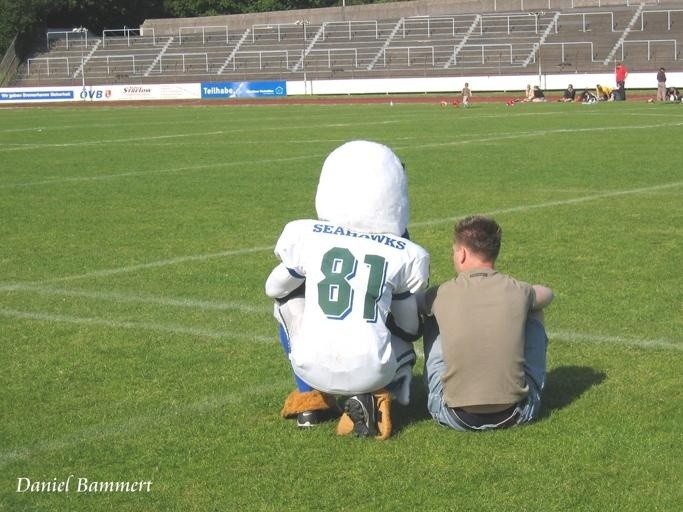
[420,216,553,433]
[265,141,429,441]
[459,82,472,109]
[525,62,679,103]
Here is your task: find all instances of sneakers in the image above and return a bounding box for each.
[296,411,318,427]
[344,393,378,437]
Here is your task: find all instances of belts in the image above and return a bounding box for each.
[445,398,527,431]
